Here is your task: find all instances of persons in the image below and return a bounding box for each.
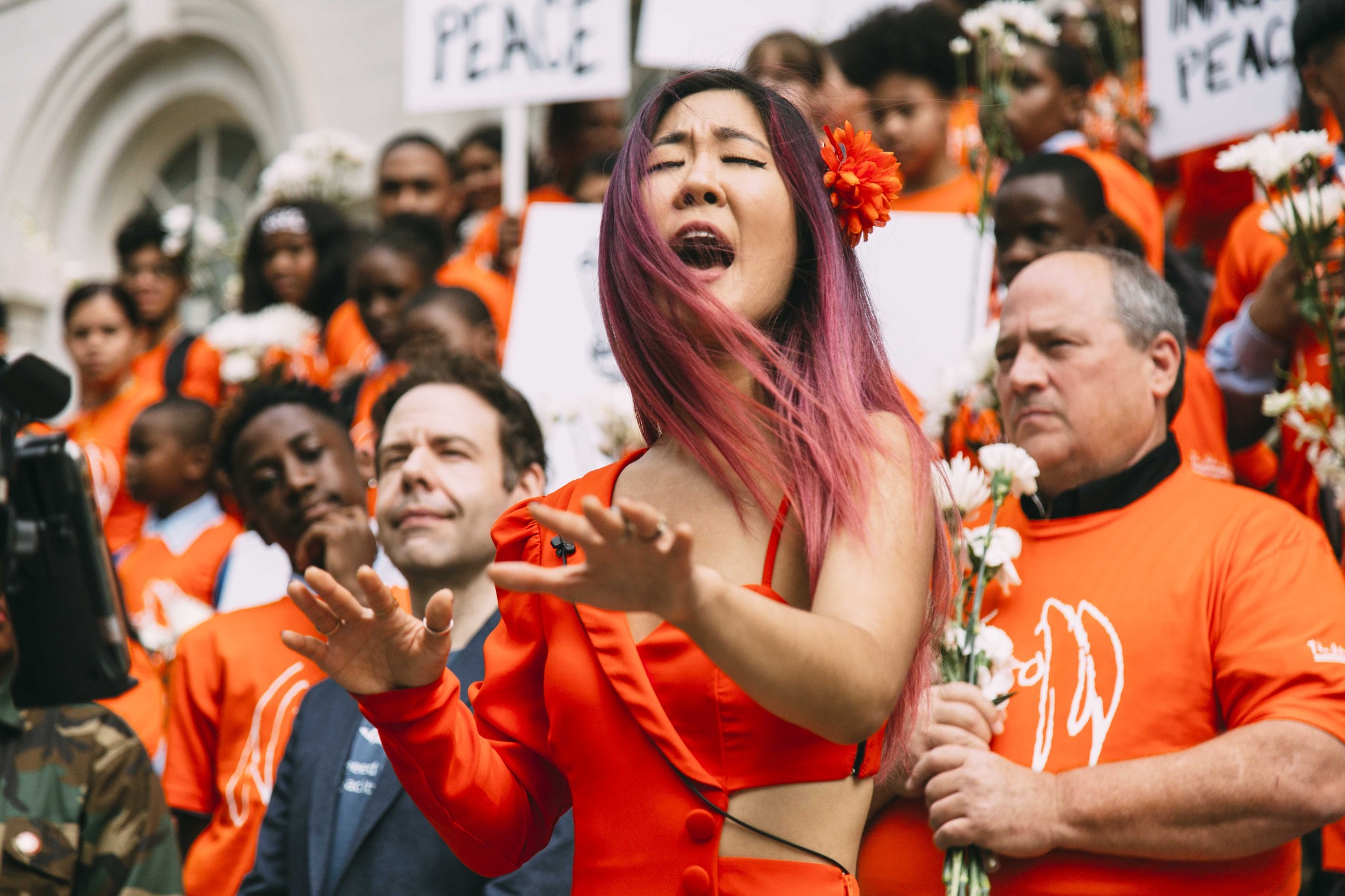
[744,0,1345,896]
[0,92,631,896]
[282,69,950,896]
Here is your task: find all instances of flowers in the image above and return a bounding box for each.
[1264,379,1345,559]
[943,0,1069,345]
[915,441,1038,896]
[212,301,327,385]
[820,119,900,252]
[1213,127,1345,416]
[253,127,382,213]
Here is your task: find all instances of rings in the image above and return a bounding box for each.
[374,596,399,620]
[423,617,453,637]
[639,522,665,543]
[314,620,340,636]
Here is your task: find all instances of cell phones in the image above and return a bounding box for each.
[0,440,136,708]
